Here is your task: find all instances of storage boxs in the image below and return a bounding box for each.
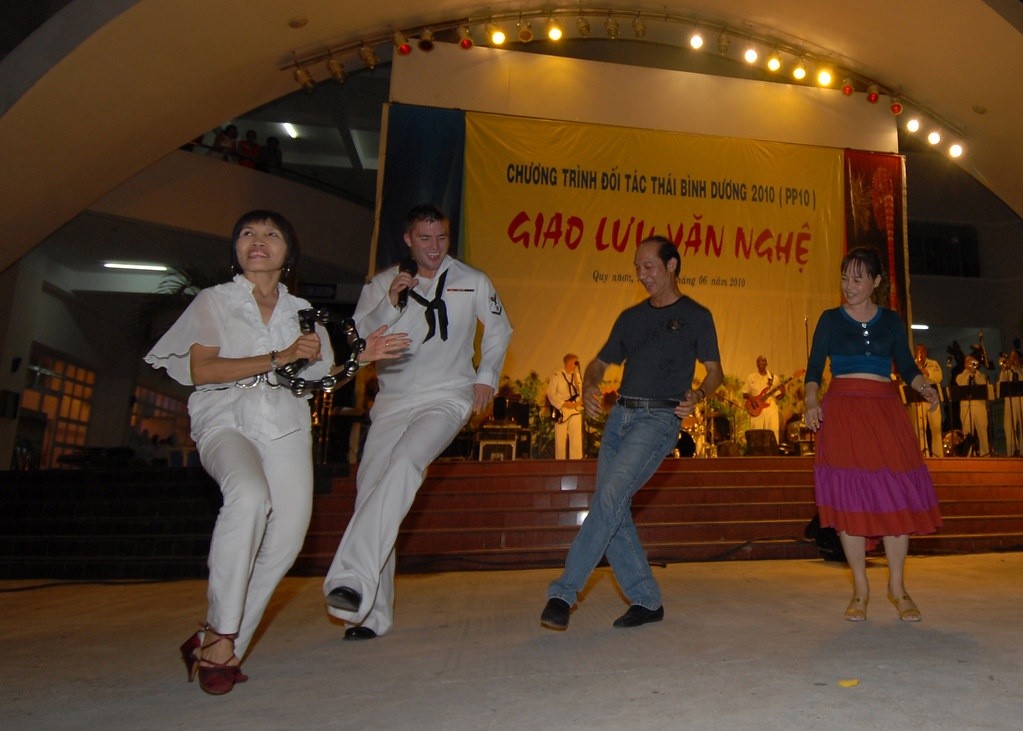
[479,425,523,459]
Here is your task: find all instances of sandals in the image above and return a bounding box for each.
[845,593,869,621]
[888,591,922,622]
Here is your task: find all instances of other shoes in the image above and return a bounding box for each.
[541,598,570,629]
[613,605,664,627]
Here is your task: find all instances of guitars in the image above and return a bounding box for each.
[744,376,795,417]
[561,398,585,423]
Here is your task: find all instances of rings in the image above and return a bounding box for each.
[386,341,389,346]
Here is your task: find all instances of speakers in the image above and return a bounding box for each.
[493,397,531,458]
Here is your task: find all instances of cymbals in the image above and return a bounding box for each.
[274,307,368,398]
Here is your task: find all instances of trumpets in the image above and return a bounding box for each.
[999,357,1021,370]
[969,359,979,368]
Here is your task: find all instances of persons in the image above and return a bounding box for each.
[181,133,203,151]
[956,355,990,458]
[742,355,786,445]
[801,247,947,622]
[546,353,584,459]
[208,125,238,161]
[257,137,282,174]
[321,202,513,642]
[239,128,259,167]
[999,351,1023,458]
[540,235,725,630]
[141,209,412,693]
[908,344,944,459]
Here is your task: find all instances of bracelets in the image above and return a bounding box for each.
[270,351,277,370]
[698,389,705,398]
[919,383,930,394]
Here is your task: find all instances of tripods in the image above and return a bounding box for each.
[903,382,939,459]
[949,334,1023,458]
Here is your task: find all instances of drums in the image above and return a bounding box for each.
[706,412,734,446]
[670,430,696,458]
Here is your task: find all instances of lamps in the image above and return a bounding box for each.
[712,27,905,115]
[293,66,316,92]
[418,28,436,54]
[544,14,563,40]
[357,42,382,66]
[630,14,648,40]
[602,12,620,38]
[390,28,413,56]
[323,55,351,84]
[514,15,535,44]
[456,23,474,50]
[573,12,590,36]
[481,19,505,45]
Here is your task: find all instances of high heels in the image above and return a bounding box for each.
[199,623,239,694]
[181,630,250,682]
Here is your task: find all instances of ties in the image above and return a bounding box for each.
[968,375,974,385]
[408,267,448,343]
[1011,369,1018,381]
[562,372,577,398]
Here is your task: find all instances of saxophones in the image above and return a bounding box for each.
[917,350,923,369]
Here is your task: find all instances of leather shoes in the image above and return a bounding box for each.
[345,627,375,639]
[326,586,360,612]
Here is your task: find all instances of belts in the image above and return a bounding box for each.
[619,397,679,408]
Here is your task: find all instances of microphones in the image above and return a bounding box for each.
[980,332,983,342]
[575,361,580,366]
[999,352,1008,357]
[397,258,418,313]
